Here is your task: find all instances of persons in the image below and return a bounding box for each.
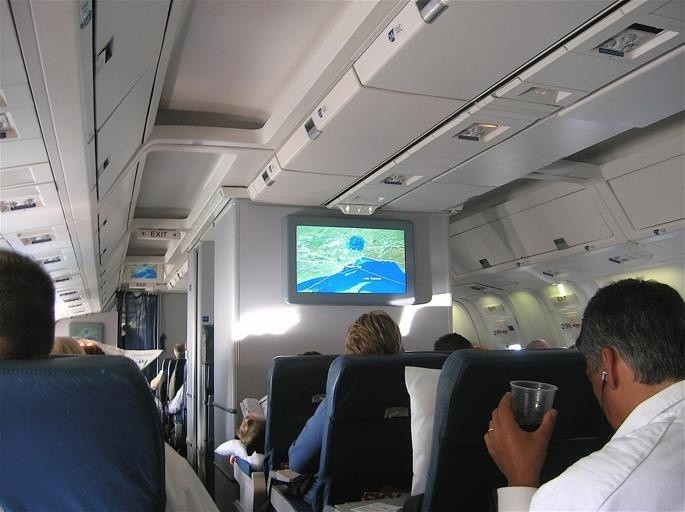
[121,324,144,349]
[1,248,103,359]
[288,310,549,502]
[151,344,183,414]
[230,352,320,464]
[484,278,685,511]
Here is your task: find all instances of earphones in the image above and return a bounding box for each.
[602,370,608,381]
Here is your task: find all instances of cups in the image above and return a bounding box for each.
[509,380,559,433]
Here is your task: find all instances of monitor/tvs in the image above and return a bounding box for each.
[69,322,103,342]
[281,216,416,305]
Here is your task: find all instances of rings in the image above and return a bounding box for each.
[488,428,493,431]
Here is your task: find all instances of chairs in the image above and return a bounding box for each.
[0,346,616,511]
